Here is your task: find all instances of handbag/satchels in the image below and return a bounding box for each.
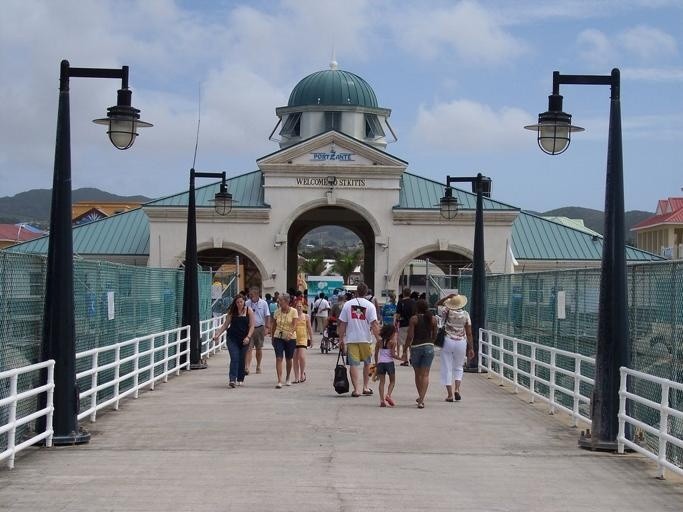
[333,365,349,394]
[396,310,406,322]
[314,307,318,314]
[434,326,444,347]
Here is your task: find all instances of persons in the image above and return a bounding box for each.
[374,324,402,408]
[221,286,355,389]
[337,282,381,397]
[435,293,475,403]
[402,299,437,409]
[365,288,431,366]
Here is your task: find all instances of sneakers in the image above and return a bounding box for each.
[415,397,425,407]
[292,380,299,383]
[256,365,263,374]
[285,375,292,386]
[381,402,387,407]
[400,361,408,366]
[385,395,395,407]
[276,383,282,388]
[236,381,244,386]
[245,368,249,375]
[300,372,306,382]
[229,381,236,388]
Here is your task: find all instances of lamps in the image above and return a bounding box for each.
[327,175,336,189]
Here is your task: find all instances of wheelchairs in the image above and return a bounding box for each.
[319,317,338,353]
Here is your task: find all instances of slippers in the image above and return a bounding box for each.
[352,391,359,397]
[363,388,374,394]
[445,399,453,402]
[455,392,461,400]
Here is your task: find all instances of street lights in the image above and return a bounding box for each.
[533,68,634,452]
[178,168,240,371]
[437,172,491,374]
[30,58,152,447]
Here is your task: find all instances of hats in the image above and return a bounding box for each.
[444,294,467,310]
